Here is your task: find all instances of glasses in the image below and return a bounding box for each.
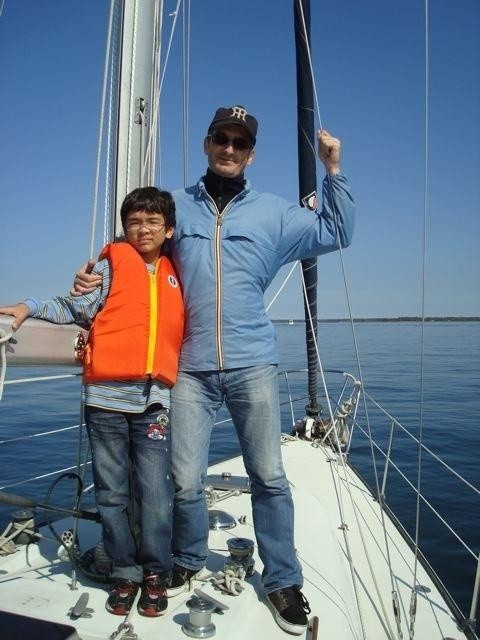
[207,132,254,151]
[123,220,169,231]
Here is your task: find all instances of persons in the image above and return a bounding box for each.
[68,104,356,635]
[0,186,186,619]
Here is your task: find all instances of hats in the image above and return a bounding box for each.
[207,105,260,140]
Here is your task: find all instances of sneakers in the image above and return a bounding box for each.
[104,577,137,616]
[137,564,206,617]
[267,586,311,635]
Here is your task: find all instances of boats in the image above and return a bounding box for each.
[288,320,294,325]
